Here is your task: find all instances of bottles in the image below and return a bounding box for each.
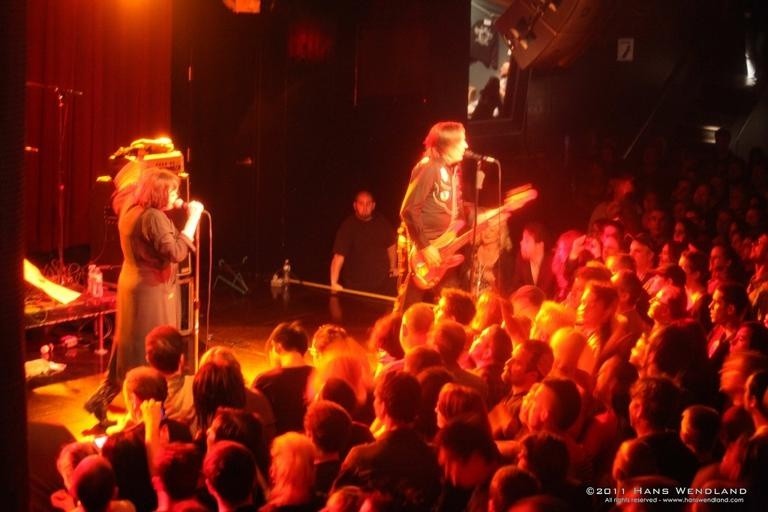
[62,335,84,350]
[87,265,104,298]
[40,342,55,353]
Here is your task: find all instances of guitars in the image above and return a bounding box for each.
[409,186,539,290]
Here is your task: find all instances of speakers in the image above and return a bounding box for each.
[494,0,607,70]
[104,172,193,281]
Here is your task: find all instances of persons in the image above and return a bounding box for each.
[50,119,768,512]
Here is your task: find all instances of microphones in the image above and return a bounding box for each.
[173,197,209,215]
[40,343,66,354]
[464,149,497,165]
[25,79,85,99]
